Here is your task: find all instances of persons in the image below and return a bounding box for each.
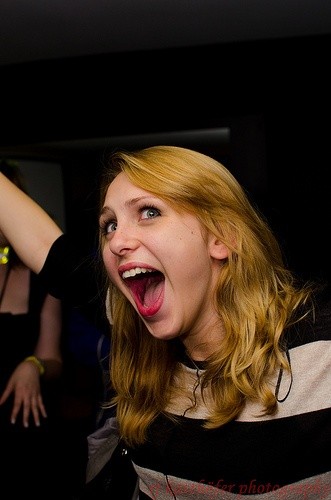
[0,145,330,500]
[0,232,67,433]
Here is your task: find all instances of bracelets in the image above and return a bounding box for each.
[25,355,47,375]
